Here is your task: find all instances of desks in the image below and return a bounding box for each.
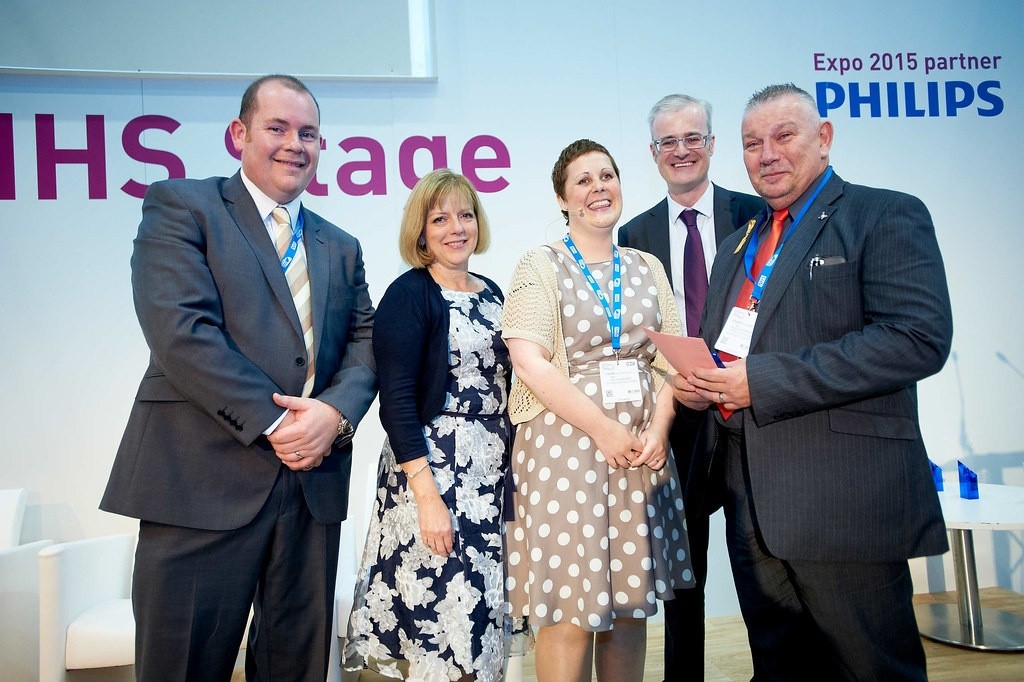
[904,481,1024,654]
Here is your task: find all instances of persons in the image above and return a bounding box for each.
[338,169,535,682]
[619,93,768,682]
[500,139,682,682]
[673,82,953,682]
[100,74,376,682]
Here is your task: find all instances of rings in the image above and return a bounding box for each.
[428,545,434,548]
[655,459,659,462]
[296,452,302,459]
[719,393,725,403]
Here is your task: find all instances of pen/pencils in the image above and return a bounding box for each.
[711,351,726,368]
[809,256,820,281]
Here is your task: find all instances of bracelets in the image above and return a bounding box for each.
[406,462,429,479]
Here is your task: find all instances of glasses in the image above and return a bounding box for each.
[655,134,712,154]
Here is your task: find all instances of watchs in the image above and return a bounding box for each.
[338,413,354,435]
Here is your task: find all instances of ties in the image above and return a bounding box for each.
[714,208,789,422]
[679,209,712,338]
[271,207,316,397]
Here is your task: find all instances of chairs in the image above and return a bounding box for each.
[0,490,360,682]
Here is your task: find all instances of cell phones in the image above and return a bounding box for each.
[816,255,846,266]
